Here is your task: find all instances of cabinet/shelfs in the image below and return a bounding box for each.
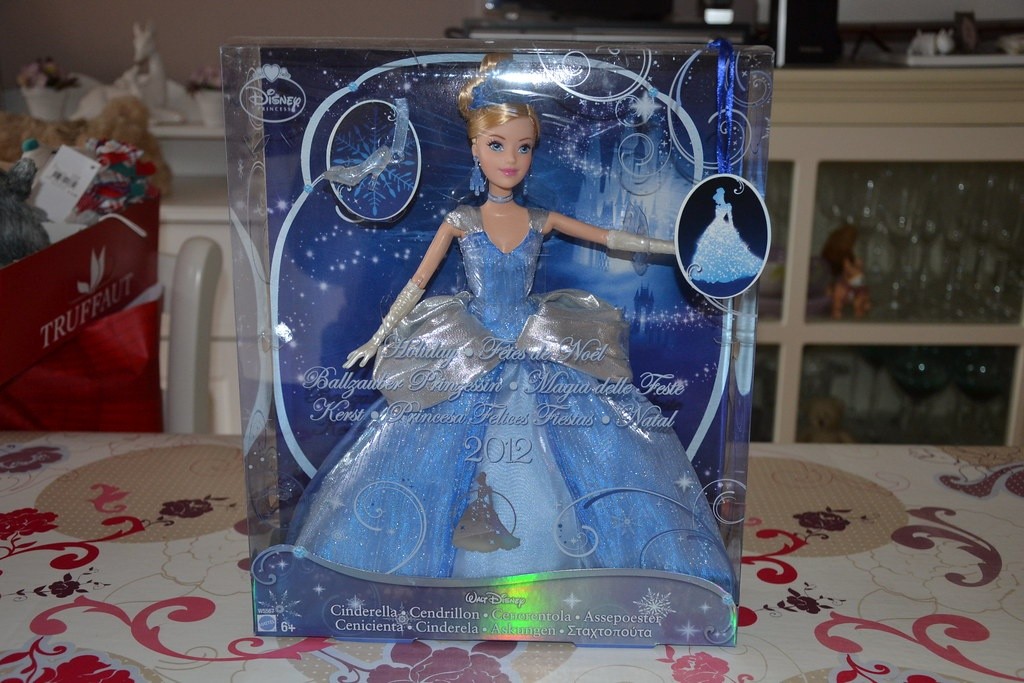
[149,121,243,440]
[146,68,1024,449]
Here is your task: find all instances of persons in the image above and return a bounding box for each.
[339,56,675,577]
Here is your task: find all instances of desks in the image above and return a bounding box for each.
[0,427,1023,683]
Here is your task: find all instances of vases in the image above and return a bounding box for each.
[21,87,69,122]
[196,89,224,129]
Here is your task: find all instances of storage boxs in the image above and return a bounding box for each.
[221,37,775,652]
[0,199,164,390]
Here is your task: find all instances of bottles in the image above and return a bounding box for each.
[22,138,52,169]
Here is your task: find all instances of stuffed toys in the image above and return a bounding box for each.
[797,395,853,443]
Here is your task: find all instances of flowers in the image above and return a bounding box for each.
[190,63,222,90]
[15,54,78,91]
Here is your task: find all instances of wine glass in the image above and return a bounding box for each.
[941,344,1015,445]
[869,345,951,446]
[819,161,1023,321]
